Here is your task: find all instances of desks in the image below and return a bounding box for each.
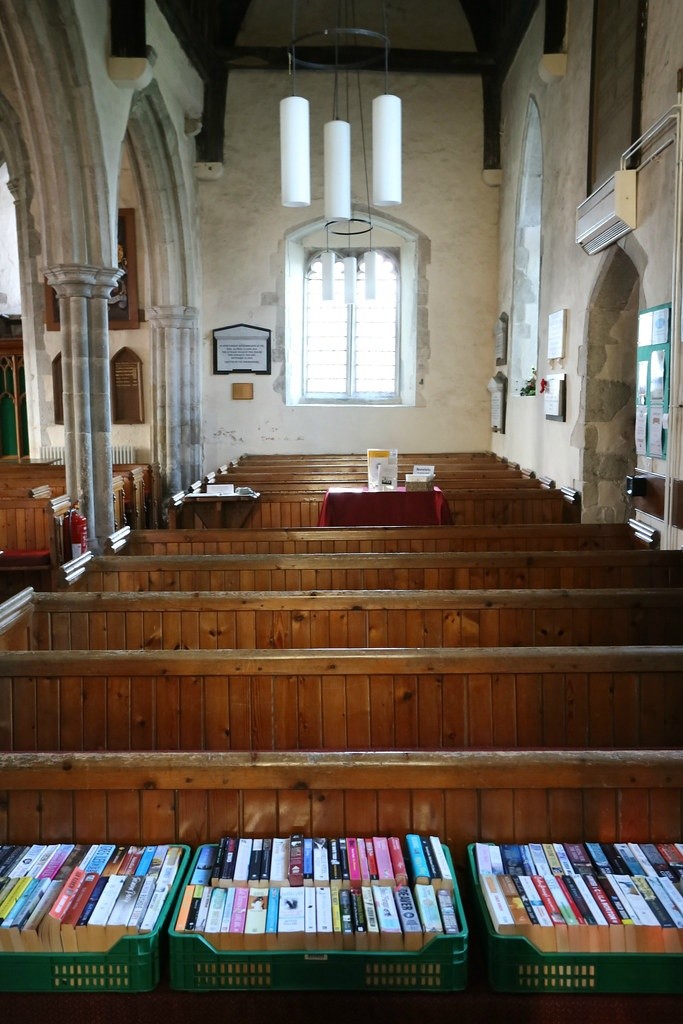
[318,484,454,527]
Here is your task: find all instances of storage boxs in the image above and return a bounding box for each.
[169,844,469,991]
[467,840,683,996]
[0,844,191,992]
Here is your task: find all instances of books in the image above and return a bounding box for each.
[0,828,682,988]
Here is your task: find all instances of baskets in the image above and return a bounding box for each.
[0,838,683,993]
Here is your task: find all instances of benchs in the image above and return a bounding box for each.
[1,452,683,845]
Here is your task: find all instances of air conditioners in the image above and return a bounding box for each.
[575,170,636,257]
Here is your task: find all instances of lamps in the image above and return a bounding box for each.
[280,0,402,221]
[322,0,377,304]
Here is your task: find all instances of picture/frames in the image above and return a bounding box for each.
[44,209,140,331]
